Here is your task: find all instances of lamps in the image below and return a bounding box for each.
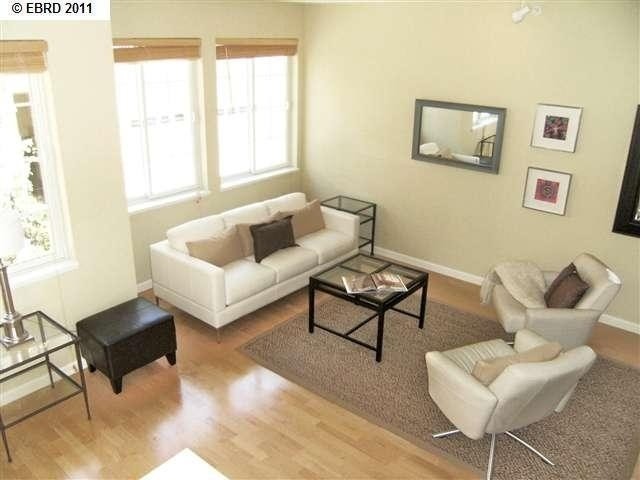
[511,0,531,24]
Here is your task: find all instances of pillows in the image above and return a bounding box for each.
[470,343,562,385]
[187,197,325,265]
[544,263,589,309]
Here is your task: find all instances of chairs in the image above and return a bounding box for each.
[482,254,622,348]
[425,328,597,480]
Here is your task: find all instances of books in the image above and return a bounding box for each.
[342,271,409,297]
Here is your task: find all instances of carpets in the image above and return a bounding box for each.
[233,285,640,480]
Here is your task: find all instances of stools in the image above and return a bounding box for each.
[79,298,177,395]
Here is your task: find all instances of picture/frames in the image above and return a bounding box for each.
[530,102,582,153]
[521,165,573,216]
[611,104,640,240]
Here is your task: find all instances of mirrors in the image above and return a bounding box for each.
[410,99,507,174]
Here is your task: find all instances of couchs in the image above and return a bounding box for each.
[150,192,358,345]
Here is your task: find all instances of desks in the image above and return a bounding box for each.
[322,194,376,256]
[309,253,429,362]
[0,312,93,463]
